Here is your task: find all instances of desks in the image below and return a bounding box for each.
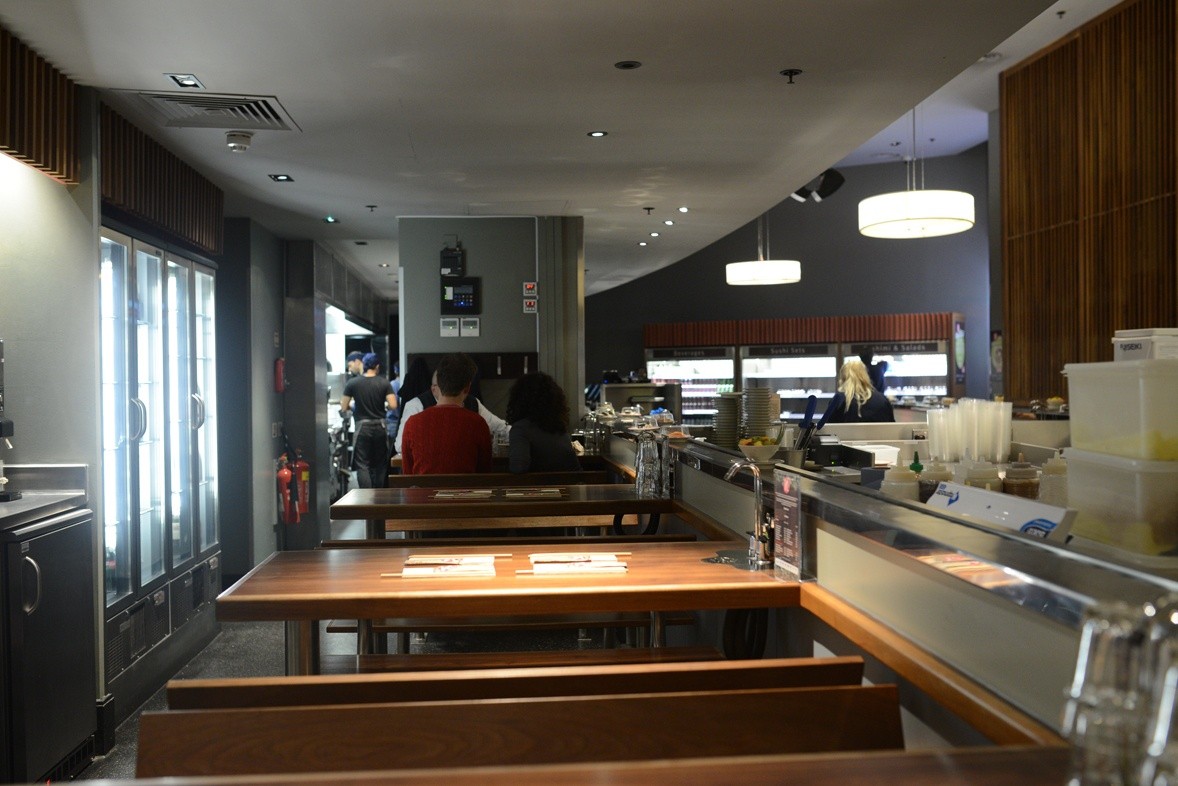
[214,543,802,680]
[390,444,508,463]
[330,481,675,539]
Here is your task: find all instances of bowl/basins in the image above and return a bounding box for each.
[740,444,780,463]
[773,447,810,470]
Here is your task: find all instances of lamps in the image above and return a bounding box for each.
[861,106,975,240]
[725,212,801,286]
[791,187,812,203]
[811,169,845,203]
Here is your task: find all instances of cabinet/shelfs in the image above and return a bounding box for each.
[0,507,98,786]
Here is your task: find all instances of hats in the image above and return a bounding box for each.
[394,361,399,374]
[363,352,378,370]
[347,351,364,361]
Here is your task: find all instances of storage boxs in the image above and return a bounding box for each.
[1064,358,1178,462]
[1063,447,1178,556]
[926,479,1080,546]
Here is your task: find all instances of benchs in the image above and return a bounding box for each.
[66,472,1069,786]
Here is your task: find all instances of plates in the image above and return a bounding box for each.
[597,415,694,443]
[710,388,778,449]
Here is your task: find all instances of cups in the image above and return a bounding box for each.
[1063,614,1178,786]
[635,441,659,494]
[925,397,1014,463]
[994,393,1005,402]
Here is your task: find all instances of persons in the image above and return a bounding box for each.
[822,346,894,423]
[507,372,573,537]
[340,351,511,539]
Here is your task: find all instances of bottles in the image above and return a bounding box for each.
[880,450,1068,510]
[584,417,595,453]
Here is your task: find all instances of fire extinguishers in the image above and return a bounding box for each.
[291,450,310,513]
[277,455,301,524]
